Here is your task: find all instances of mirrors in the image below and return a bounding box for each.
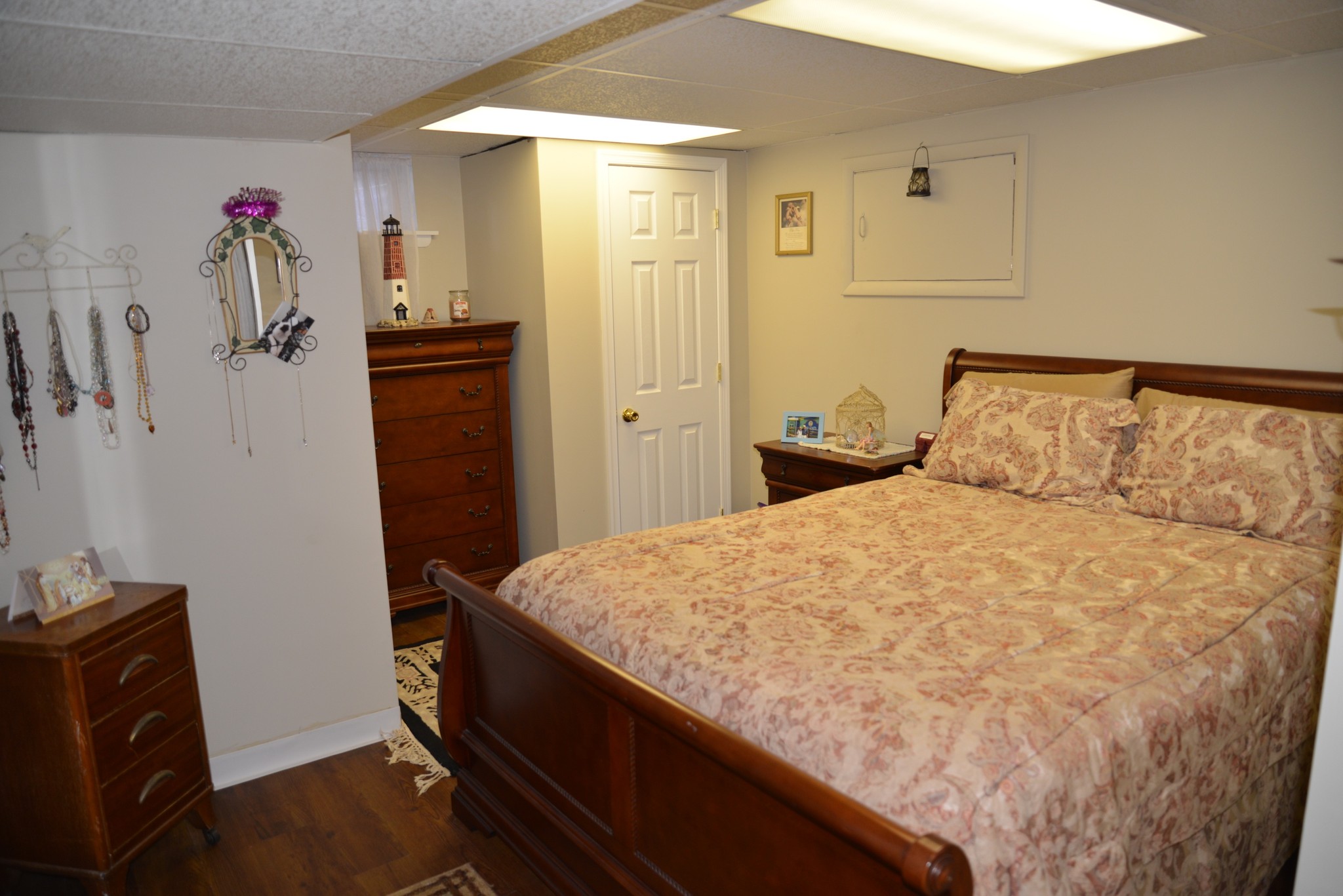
[199,213,318,370]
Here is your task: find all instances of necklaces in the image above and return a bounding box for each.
[205,275,220,364]
[131,306,155,434]
[297,364,307,447]
[241,370,252,458]
[48,308,82,417]
[82,306,121,449]
[2,311,40,491]
[224,359,236,444]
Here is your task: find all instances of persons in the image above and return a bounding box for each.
[796,425,808,438]
[854,422,874,452]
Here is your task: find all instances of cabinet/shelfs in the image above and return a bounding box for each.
[364,317,521,627]
[0,579,223,896]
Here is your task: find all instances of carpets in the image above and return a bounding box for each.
[386,861,499,896]
[383,635,462,797]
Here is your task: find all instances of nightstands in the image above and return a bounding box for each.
[752,431,928,508]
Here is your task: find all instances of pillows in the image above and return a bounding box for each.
[943,367,1135,401]
[1085,403,1343,554]
[1131,387,1343,434]
[902,377,1141,508]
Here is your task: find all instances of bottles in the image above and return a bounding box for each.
[449,290,471,321]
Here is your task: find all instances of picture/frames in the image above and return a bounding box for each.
[774,191,814,256]
[780,409,826,445]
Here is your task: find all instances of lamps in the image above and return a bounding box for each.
[905,145,931,197]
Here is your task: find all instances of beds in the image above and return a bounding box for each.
[417,346,1343,896]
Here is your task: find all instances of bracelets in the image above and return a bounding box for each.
[125,304,150,333]
